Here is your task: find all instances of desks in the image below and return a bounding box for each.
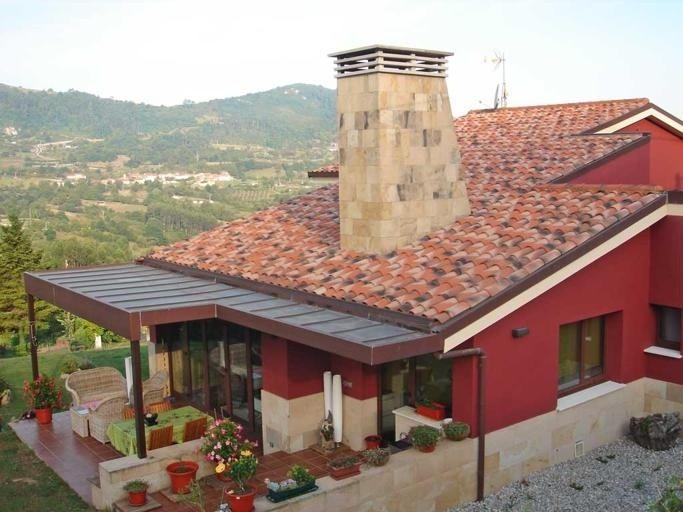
[108,404,216,456]
[69,398,106,437]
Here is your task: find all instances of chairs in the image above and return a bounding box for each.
[213,340,262,390]
[87,399,124,444]
[182,415,208,444]
[145,423,173,448]
[146,400,171,415]
[144,367,167,388]
[141,383,165,404]
[120,403,148,420]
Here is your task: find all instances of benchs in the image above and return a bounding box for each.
[64,366,127,405]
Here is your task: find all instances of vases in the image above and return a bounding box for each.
[224,483,257,512]
[363,433,382,448]
[265,480,318,500]
[33,404,53,424]
[373,455,389,467]
[213,454,238,482]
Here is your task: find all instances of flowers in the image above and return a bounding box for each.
[361,447,390,471]
[20,371,66,411]
[261,465,317,490]
[194,416,258,464]
[215,450,259,491]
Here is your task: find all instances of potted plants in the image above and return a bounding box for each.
[413,398,448,419]
[408,424,439,452]
[164,459,199,493]
[120,479,151,506]
[326,454,362,480]
[443,420,471,441]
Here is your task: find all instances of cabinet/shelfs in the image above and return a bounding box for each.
[391,405,452,443]
